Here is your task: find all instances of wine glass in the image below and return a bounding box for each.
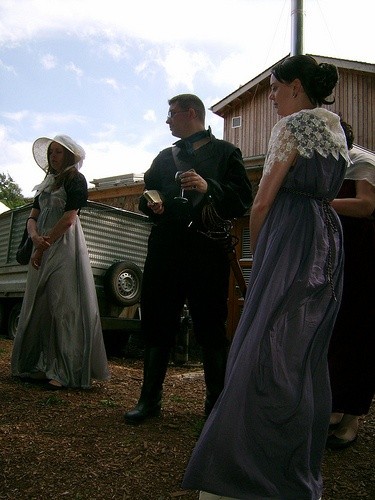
[172,171,188,203]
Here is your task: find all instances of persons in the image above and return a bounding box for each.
[125,95,253,422]
[326,120,375,449]
[182,56,350,500]
[12,134,110,389]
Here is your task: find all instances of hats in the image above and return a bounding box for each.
[32,134,85,173]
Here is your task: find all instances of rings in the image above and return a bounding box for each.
[191,186,197,191]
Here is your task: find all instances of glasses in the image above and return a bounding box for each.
[169,110,189,118]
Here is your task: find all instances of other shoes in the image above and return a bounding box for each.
[325,431,358,448]
[328,414,345,430]
[44,380,65,390]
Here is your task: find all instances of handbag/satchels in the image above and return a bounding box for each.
[16,217,37,265]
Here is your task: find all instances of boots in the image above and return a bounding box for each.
[124,346,170,422]
[194,351,227,440]
